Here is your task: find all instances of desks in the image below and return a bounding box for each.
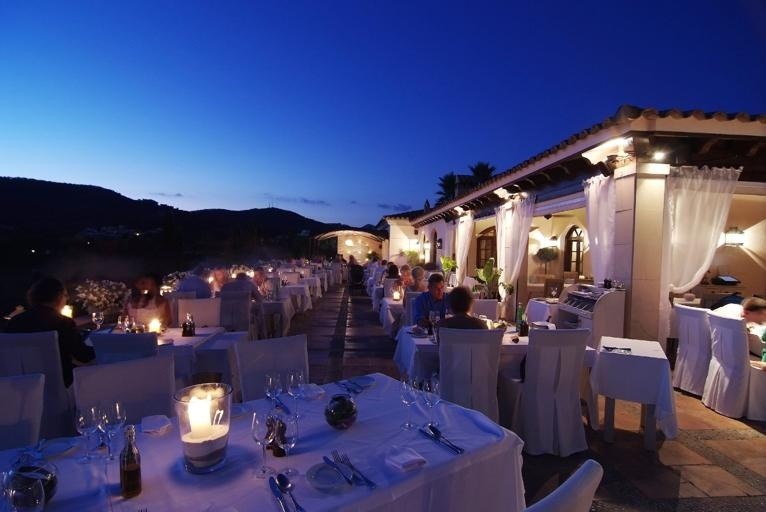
[594,334,668,452]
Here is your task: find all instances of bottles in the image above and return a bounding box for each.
[516,308,523,330]
[119,424,142,498]
[182,313,196,336]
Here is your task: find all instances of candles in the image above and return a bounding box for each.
[393,291,401,298]
[188,397,215,436]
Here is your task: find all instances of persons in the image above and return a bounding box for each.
[412,273,451,328]
[436,285,487,346]
[208,265,232,292]
[373,260,387,280]
[393,265,413,299]
[403,266,428,308]
[220,273,266,332]
[333,254,342,263]
[713,297,766,358]
[175,265,211,298]
[120,273,173,334]
[251,271,266,301]
[347,255,359,272]
[3,277,96,370]
[381,262,394,284]
[367,256,379,271]
[385,265,400,279]
[340,254,347,263]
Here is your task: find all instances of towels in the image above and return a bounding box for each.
[141,414,172,437]
[385,444,427,473]
[414,337,434,345]
[297,383,326,400]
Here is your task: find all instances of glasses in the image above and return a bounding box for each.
[63,294,70,299]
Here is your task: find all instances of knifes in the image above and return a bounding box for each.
[322,455,353,487]
[418,428,460,456]
[268,476,289,512]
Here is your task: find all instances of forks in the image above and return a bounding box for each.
[331,447,377,490]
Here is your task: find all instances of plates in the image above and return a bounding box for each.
[306,461,343,488]
[231,402,254,420]
[351,375,376,387]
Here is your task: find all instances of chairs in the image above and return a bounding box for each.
[706,311,766,420]
[672,303,710,395]
[71,353,174,424]
[281,272,301,285]
[1,331,67,408]
[164,292,198,324]
[471,299,499,322]
[405,290,422,323]
[176,298,221,326]
[231,335,309,401]
[0,374,45,446]
[519,461,604,512]
[430,327,502,422]
[384,278,403,296]
[88,332,157,363]
[217,290,250,331]
[503,327,589,457]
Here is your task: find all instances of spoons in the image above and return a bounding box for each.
[428,425,465,453]
[275,473,304,512]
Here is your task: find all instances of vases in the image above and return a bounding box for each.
[90,309,107,332]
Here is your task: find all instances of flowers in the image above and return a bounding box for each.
[544,278,565,299]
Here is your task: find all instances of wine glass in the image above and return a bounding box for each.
[275,418,299,478]
[251,410,275,478]
[74,406,100,465]
[429,311,440,325]
[0,474,44,512]
[16,459,62,512]
[263,371,282,418]
[399,374,419,431]
[422,377,440,428]
[98,401,126,464]
[92,312,105,330]
[286,369,304,422]
